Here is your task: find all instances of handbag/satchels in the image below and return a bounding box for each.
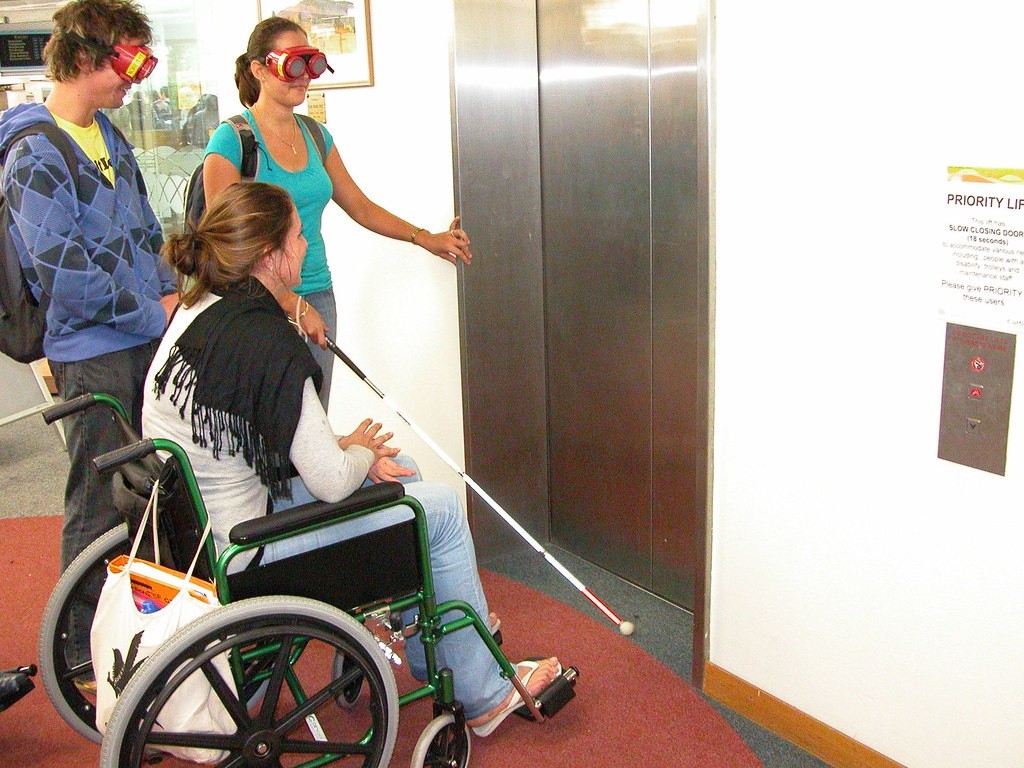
[93,491,241,762]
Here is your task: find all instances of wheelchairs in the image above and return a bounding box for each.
[40,393,579,768]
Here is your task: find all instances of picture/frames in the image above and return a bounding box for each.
[257,0,376,92]
[1,31,56,70]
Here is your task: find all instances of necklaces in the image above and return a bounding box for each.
[256,105,297,154]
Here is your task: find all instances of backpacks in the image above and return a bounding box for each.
[182,113,327,245]
[0,119,128,363]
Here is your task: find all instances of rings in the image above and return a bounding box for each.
[451,231,454,235]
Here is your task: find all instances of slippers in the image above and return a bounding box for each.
[473,660,562,737]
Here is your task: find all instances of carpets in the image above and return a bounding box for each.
[0,515,761,768]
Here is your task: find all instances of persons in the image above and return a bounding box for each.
[142,182,562,737]
[126,85,170,130]
[0,0,180,695]
[202,16,472,416]
[181,93,219,148]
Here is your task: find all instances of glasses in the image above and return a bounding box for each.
[258,44,334,84]
[59,26,158,84]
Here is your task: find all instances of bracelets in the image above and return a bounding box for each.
[298,302,309,317]
[411,228,425,245]
